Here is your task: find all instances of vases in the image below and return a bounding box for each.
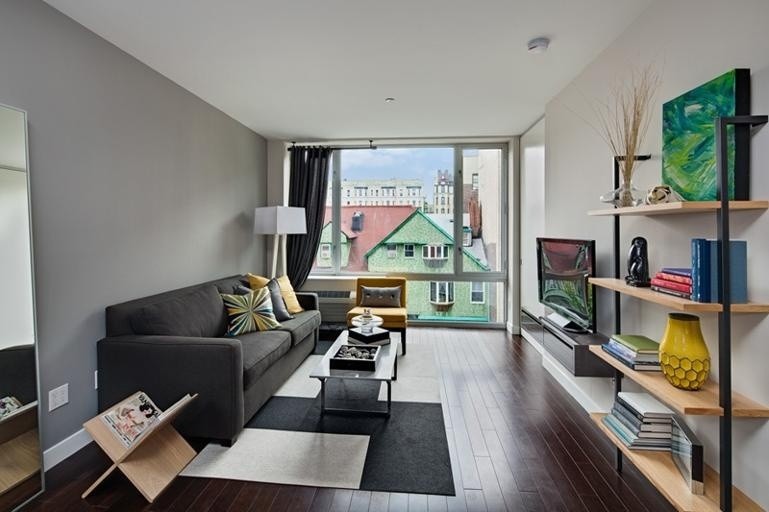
[599,181,648,208]
[658,313,712,391]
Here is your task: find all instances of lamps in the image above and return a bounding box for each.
[251,206,306,279]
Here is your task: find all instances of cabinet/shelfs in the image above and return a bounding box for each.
[586,200,769,511]
[518,306,627,376]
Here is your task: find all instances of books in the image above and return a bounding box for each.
[601,391,675,452]
[601,334,662,372]
[101,391,192,451]
[0,397,25,419]
[651,238,748,304]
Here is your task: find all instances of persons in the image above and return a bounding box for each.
[115,402,155,436]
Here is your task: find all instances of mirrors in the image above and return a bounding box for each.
[0,103,46,512]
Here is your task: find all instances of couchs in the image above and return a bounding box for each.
[346,277,408,356]
[96,274,322,447]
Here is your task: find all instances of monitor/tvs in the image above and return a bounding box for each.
[535,236,598,336]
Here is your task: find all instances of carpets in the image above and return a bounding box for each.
[177,342,455,497]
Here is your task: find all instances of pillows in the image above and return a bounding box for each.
[359,285,401,307]
[220,286,283,337]
[246,273,304,314]
[232,277,296,322]
